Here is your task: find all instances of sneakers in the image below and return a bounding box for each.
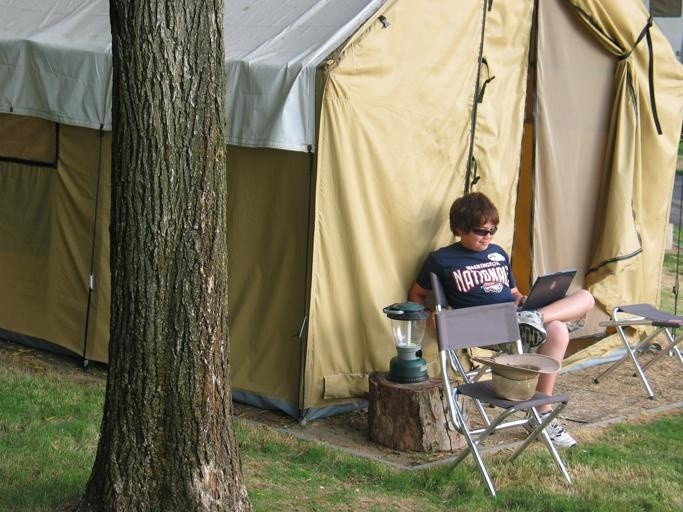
[523,408,577,449]
[516,310,547,347]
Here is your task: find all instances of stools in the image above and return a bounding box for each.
[594,304,683,399]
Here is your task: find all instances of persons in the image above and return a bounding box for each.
[408,192,595,447]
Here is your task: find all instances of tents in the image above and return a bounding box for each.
[1,0,682,426]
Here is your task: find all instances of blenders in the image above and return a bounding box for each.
[383,301,431,385]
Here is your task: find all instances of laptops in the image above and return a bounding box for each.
[516,268,577,312]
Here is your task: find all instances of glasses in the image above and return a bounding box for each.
[473,227,498,237]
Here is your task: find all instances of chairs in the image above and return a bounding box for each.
[430,270,573,498]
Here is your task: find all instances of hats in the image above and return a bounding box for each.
[472,354,561,403]
